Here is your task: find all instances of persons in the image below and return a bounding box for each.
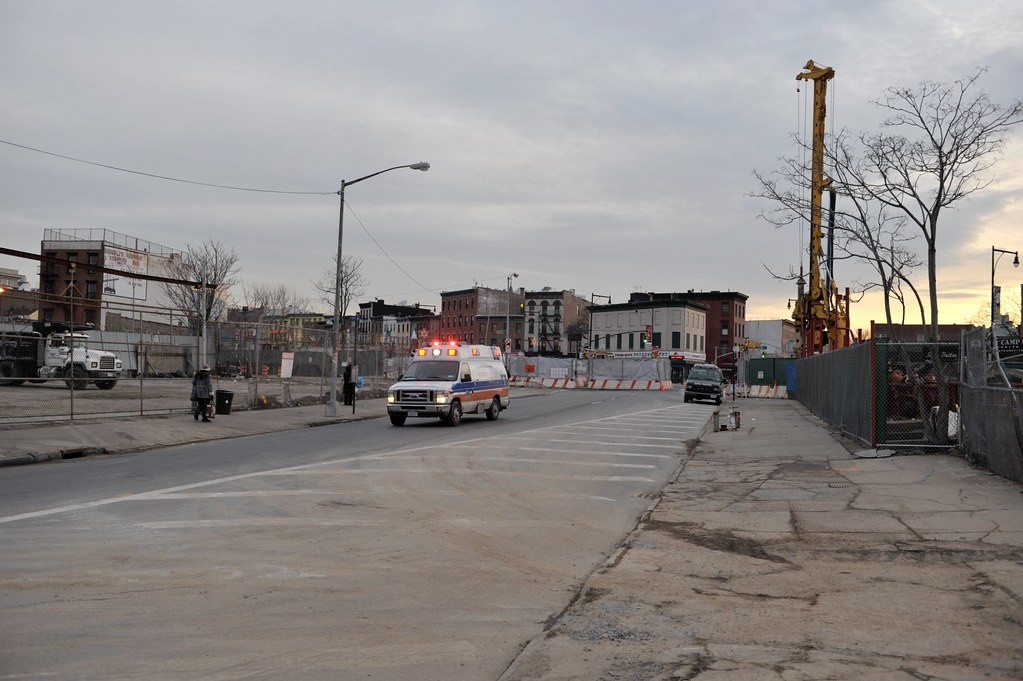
[342,363,361,406]
[192,364,214,422]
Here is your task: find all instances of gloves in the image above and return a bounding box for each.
[210,395,214,401]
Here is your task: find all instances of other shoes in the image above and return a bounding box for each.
[194,412,199,420]
[202,418,211,422]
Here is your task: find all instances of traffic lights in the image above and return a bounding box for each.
[643,335,647,342]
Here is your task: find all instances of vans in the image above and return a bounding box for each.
[387,341,510,427]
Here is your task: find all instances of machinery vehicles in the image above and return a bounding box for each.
[796,60,852,356]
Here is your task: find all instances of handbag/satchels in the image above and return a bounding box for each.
[189,389,198,402]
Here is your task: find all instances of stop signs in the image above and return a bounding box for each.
[652,346,658,354]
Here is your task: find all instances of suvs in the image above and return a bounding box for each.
[684,364,726,404]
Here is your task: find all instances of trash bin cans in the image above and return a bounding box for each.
[216,389,234,414]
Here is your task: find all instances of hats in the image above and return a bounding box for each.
[201,364,210,371]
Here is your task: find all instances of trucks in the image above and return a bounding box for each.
[0,332,123,390]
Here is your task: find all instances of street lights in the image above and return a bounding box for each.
[991,245,1019,349]
[506,272,519,370]
[589,293,611,348]
[325,161,430,418]
